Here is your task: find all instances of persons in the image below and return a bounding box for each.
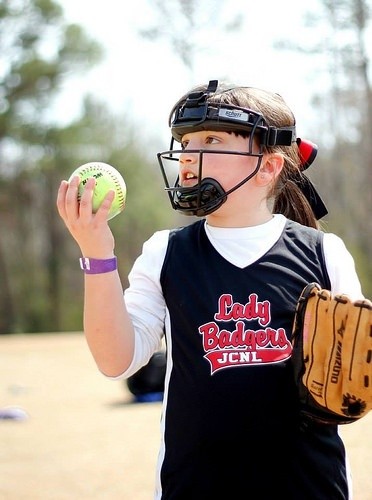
[55,80,372,499]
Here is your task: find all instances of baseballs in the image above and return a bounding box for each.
[70,161,126,217]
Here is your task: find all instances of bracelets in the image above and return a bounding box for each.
[79,255,117,275]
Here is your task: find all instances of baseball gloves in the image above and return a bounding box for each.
[288,282,372,425]
